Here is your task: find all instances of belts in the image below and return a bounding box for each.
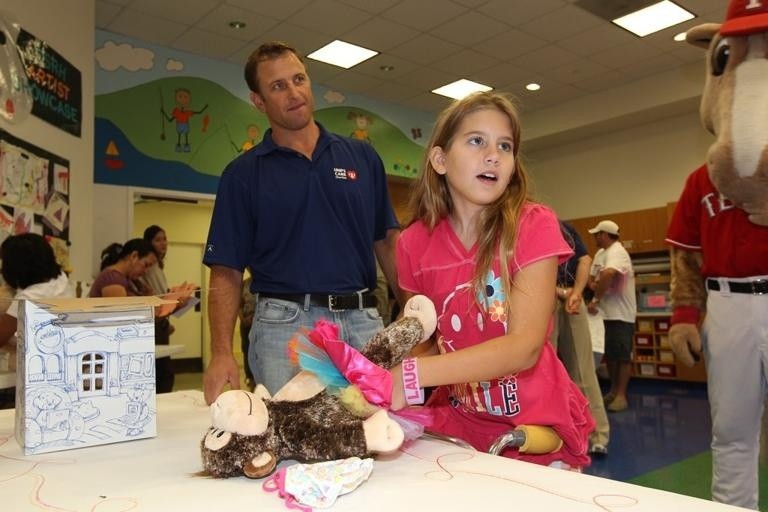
[258,291,378,309]
[707,278,768,293]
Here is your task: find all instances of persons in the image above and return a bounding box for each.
[0,225,197,392]
[375,92,596,473]
[202,40,400,404]
[552,216,637,453]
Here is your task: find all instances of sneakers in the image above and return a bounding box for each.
[590,445,608,456]
[603,393,627,410]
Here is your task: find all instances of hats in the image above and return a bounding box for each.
[588,220,620,235]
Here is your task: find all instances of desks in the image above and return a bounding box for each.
[1,386,763,512]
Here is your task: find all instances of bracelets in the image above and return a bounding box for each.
[401,355,424,405]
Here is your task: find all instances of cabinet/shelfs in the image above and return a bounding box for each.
[624,271,709,385]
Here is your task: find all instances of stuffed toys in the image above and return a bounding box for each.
[661,0,767,512]
[189,292,441,480]
